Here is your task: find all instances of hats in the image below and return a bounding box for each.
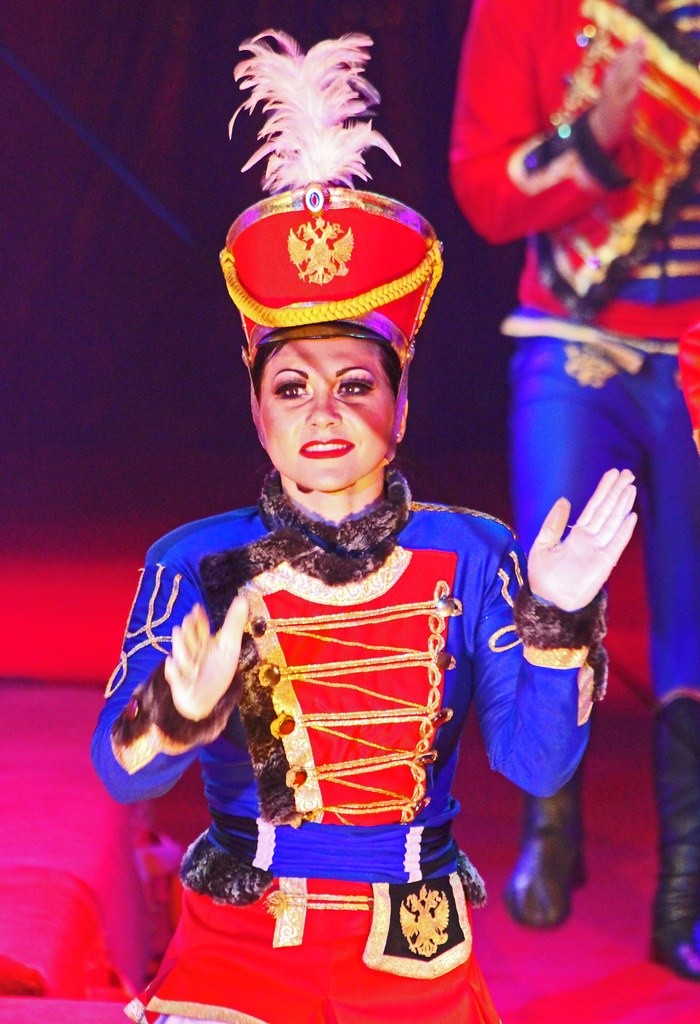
[218,29,443,376]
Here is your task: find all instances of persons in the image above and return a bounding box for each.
[88,183,639,1024]
[447,0,700,982]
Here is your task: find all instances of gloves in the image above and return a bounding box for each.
[527,467,638,613]
[164,596,251,721]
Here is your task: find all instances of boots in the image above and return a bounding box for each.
[651,686,700,981]
[507,756,586,930]
